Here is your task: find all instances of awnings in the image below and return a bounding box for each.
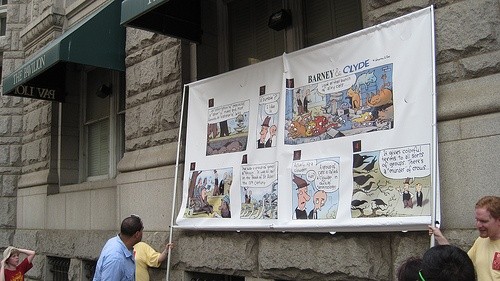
[3,0,204,105]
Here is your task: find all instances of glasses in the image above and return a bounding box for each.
[141,227,144,230]
[418,268,425,281]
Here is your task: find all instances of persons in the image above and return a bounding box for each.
[397,245,477,281]
[429,196,500,281]
[0,246,36,281]
[93,217,144,281]
[133,241,175,281]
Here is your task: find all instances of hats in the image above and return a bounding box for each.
[1,246,18,262]
